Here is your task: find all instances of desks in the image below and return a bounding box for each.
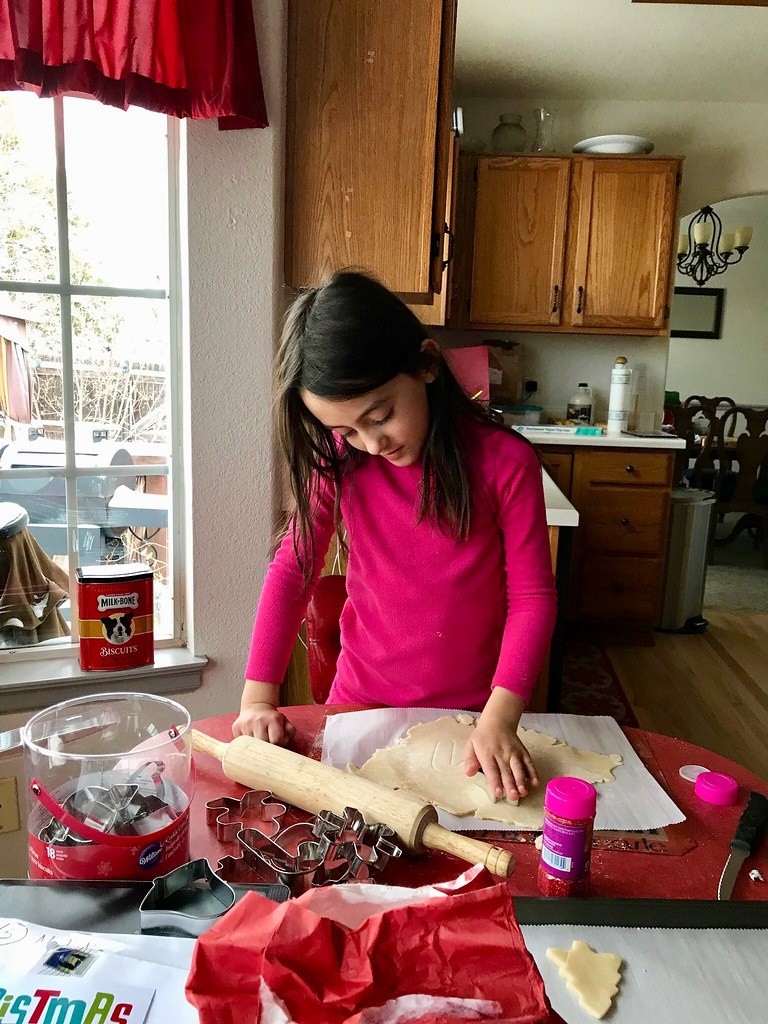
[692,441,737,460]
[110,703,768,901]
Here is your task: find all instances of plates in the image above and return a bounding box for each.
[572,133,656,154]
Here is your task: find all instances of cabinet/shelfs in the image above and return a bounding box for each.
[287,0,686,336]
[529,448,679,712]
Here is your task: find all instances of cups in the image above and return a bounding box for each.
[637,412,654,432]
[629,393,639,430]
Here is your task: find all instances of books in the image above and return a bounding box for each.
[511,425,603,438]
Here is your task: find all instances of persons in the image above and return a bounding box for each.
[230,267,558,802]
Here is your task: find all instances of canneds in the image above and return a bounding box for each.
[536,777,598,898]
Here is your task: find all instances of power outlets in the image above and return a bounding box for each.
[522,376,539,402]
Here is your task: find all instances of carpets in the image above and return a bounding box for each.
[705,512,768,613]
[557,630,640,729]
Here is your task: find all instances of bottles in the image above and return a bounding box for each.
[566,382,594,425]
[532,107,559,153]
[606,356,632,435]
[490,113,527,151]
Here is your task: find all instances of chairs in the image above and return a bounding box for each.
[663,393,768,554]
[305,575,349,707]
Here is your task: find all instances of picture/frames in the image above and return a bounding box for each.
[670,287,723,339]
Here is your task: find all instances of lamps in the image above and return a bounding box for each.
[677,206,754,288]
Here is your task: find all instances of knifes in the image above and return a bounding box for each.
[718,790,768,900]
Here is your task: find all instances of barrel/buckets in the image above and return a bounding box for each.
[21,693,196,880]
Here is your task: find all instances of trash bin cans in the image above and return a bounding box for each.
[658,488,717,633]
